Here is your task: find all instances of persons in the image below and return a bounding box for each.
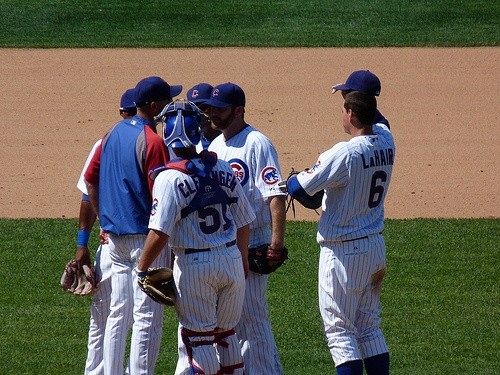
[74,76,222,375]
[286,82,395,375]
[133,113,257,375]
[207,82,289,375]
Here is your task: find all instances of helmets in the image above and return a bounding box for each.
[162,104,203,149]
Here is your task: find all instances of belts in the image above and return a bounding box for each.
[341,231,382,243]
[185,239,236,255]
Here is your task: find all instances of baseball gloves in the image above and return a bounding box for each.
[137,266,177,305]
[59,259,96,296]
[286,171,324,210]
[248,243,288,274]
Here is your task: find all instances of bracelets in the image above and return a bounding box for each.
[77,230,90,247]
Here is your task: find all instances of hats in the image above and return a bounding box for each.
[333,70,382,98]
[186,82,213,104]
[134,76,182,110]
[201,82,246,109]
[118,88,136,108]
